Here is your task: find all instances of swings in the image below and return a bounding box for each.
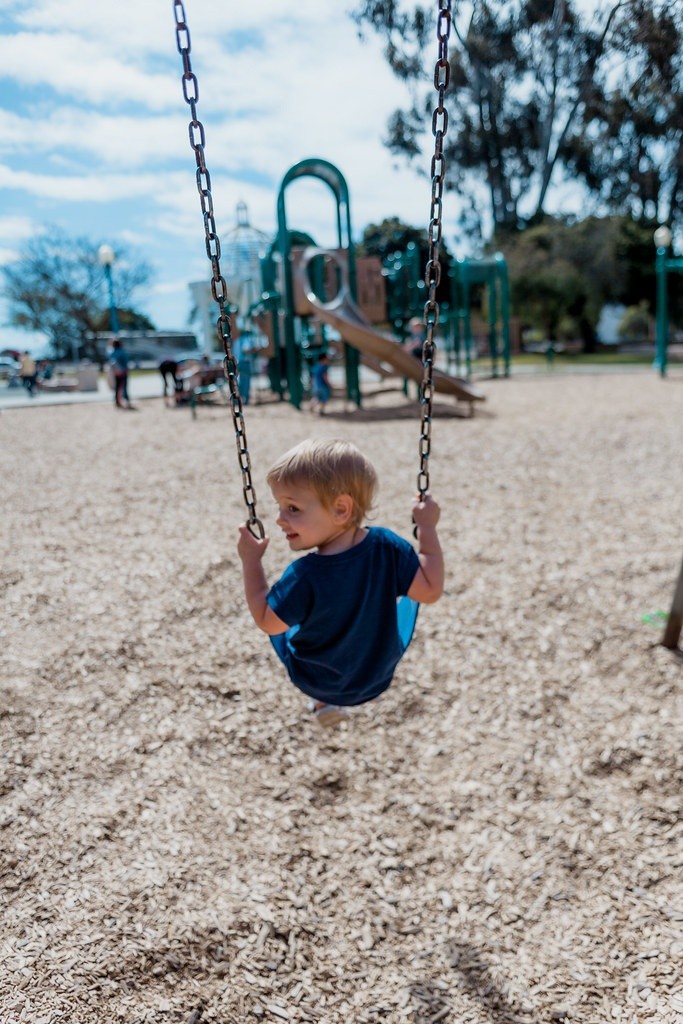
[174,0,454,666]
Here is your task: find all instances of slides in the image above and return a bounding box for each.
[305,291,491,404]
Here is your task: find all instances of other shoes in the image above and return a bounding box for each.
[307,697,349,728]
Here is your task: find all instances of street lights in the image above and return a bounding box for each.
[98,242,119,337]
[651,225,673,377]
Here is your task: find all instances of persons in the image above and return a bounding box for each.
[109,340,131,409]
[160,353,251,405]
[20,351,53,393]
[310,353,332,414]
[402,320,425,358]
[238,437,444,726]
